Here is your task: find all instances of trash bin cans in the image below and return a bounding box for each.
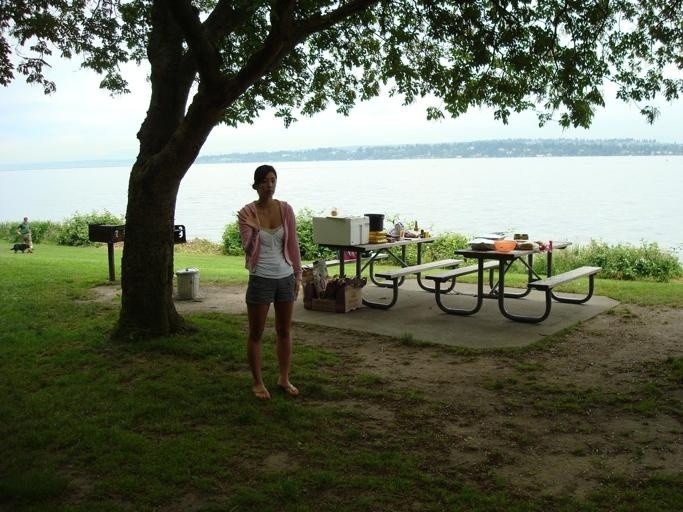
[176,269,200,299]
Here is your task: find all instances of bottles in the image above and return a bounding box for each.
[414,220,429,238]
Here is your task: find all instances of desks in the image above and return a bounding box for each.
[319,237,434,310]
[454,241,573,323]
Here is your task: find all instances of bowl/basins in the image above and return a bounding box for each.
[494,240,517,252]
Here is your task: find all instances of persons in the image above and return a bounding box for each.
[236,165,303,400]
[16,217,34,251]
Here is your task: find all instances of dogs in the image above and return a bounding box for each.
[10,244,30,253]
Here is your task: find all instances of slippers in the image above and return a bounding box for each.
[276,381,298,395]
[252,383,271,400]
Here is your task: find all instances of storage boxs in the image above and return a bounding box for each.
[313,215,370,246]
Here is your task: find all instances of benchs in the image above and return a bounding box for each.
[426,259,602,323]
[301,253,462,310]
